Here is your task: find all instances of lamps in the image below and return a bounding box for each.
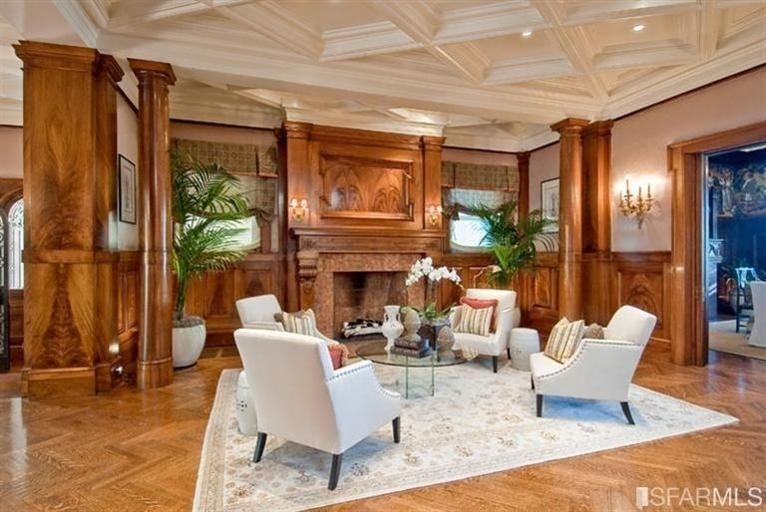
[616,177,654,229]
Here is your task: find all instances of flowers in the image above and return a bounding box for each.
[405,257,462,325]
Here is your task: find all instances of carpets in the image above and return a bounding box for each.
[707,318,765,361]
[191,351,739,512]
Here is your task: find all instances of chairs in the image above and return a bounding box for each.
[733,266,765,347]
[445,288,657,425]
[233,289,401,487]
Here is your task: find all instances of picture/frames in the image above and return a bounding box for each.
[117,153,136,225]
[539,177,559,234]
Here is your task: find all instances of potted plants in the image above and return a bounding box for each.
[167,148,250,369]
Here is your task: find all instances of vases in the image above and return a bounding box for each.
[382,305,404,355]
[417,320,450,351]
